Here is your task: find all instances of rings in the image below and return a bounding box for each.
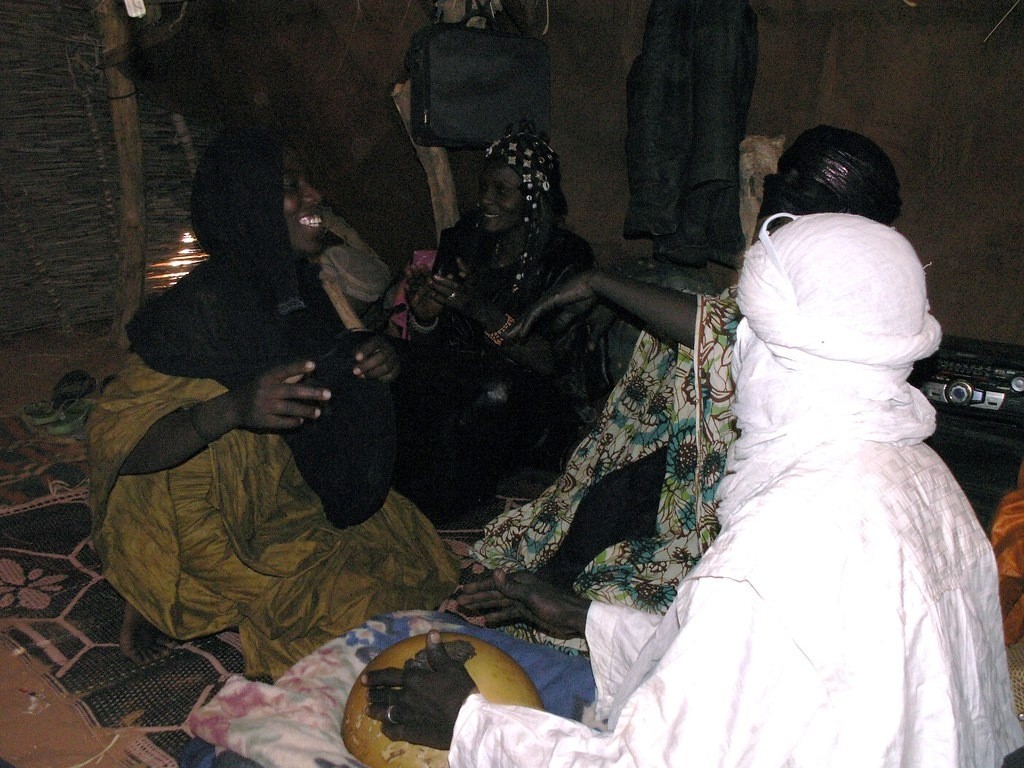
[449,292,456,299]
[386,705,398,725]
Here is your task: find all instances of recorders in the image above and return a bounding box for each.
[902,335,1023,423]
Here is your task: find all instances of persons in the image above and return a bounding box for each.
[83,125,456,682]
[480,125,898,657]
[403,131,597,521]
[359,212,1024,768]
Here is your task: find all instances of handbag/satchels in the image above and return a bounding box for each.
[404,10,552,150]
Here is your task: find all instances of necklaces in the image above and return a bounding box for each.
[495,237,505,268]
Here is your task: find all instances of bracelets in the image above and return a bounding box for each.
[485,314,516,345]
[190,406,217,441]
[411,315,439,334]
